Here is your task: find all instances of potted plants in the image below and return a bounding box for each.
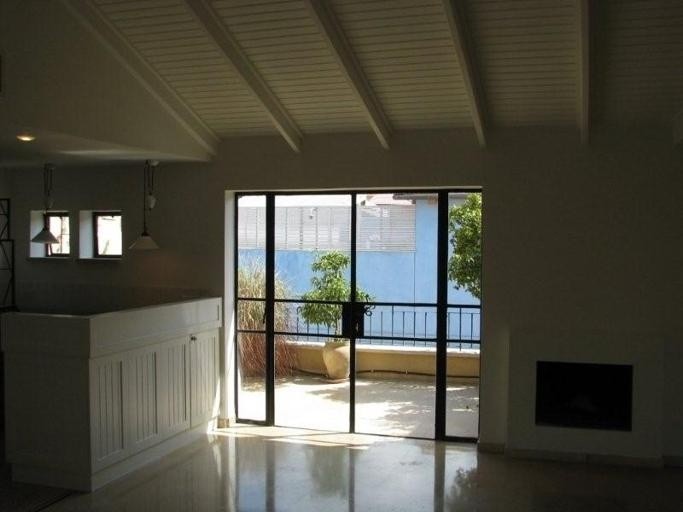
[299,251,375,378]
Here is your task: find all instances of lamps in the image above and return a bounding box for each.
[126,158,160,252]
[30,163,60,244]
